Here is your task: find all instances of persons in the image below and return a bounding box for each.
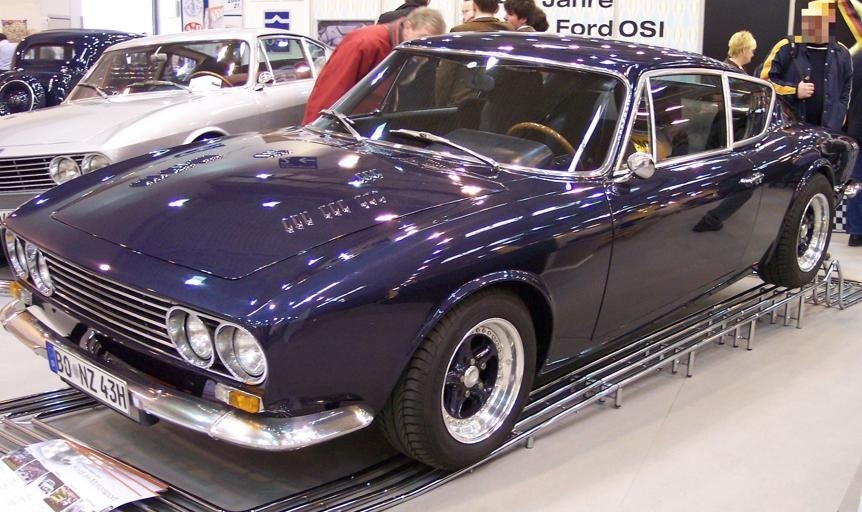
[376,1,436,110]
[841,46,862,248]
[461,0,475,22]
[755,0,855,134]
[435,0,515,107]
[503,0,536,31]
[535,7,549,31]
[300,6,445,128]
[669,92,724,158]
[722,31,758,69]
[693,188,755,234]
[0,32,19,72]
[734,88,767,141]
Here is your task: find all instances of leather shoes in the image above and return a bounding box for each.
[691,218,724,234]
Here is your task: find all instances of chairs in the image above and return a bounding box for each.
[454,66,722,167]
